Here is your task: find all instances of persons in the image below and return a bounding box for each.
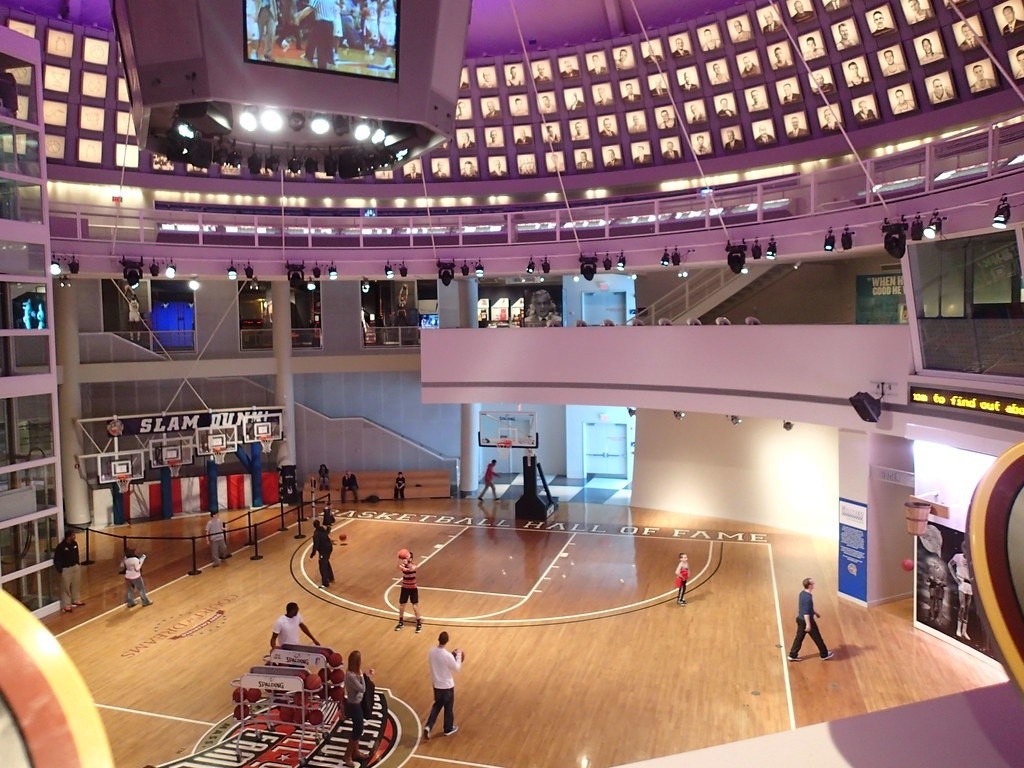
[120,547,154,607]
[394,552,422,634]
[395,283,410,325]
[269,602,321,649]
[310,520,335,588]
[701,0,1024,154]
[948,540,973,640]
[341,470,359,503]
[319,464,330,491]
[394,472,406,500]
[424,631,462,739]
[405,38,710,180]
[787,578,835,661]
[246,0,397,71]
[124,284,140,340]
[204,511,228,567]
[926,558,946,625]
[524,288,562,328]
[478,460,501,501]
[341,651,375,767]
[676,553,690,604]
[54,529,86,613]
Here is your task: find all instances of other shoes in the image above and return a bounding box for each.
[478,497,483,501]
[329,579,335,582]
[128,602,137,607]
[72,601,85,605]
[678,600,686,603]
[63,607,72,611]
[143,601,153,606]
[494,498,499,500]
[787,655,803,660]
[319,584,329,588]
[820,652,837,659]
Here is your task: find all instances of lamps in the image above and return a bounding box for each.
[659,245,683,265]
[783,419,793,431]
[383,259,409,280]
[361,278,370,292]
[526,255,552,274]
[673,411,685,420]
[726,414,743,426]
[156,104,416,180]
[434,257,484,286]
[881,209,948,259]
[990,194,1012,231]
[578,252,626,282]
[225,258,255,280]
[626,408,638,419]
[725,237,777,274]
[822,225,854,252]
[285,258,341,294]
[50,254,201,292]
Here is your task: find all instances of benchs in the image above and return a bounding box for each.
[303,471,452,503]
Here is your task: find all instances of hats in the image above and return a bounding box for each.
[125,548,136,555]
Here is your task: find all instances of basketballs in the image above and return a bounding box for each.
[318,684,344,701]
[293,692,309,705]
[293,672,322,690]
[308,709,324,725]
[331,669,344,685]
[902,558,914,571]
[399,548,410,560]
[279,706,312,724]
[451,649,465,663]
[318,667,331,682]
[232,687,262,703]
[234,704,250,719]
[339,533,347,541]
[319,648,342,667]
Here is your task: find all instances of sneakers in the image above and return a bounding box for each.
[443,726,458,735]
[395,621,404,631]
[425,726,430,739]
[415,625,422,632]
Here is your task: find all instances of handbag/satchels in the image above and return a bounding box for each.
[360,673,375,719]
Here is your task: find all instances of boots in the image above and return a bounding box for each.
[353,739,370,759]
[343,740,361,767]
[956,621,962,637]
[962,623,971,640]
